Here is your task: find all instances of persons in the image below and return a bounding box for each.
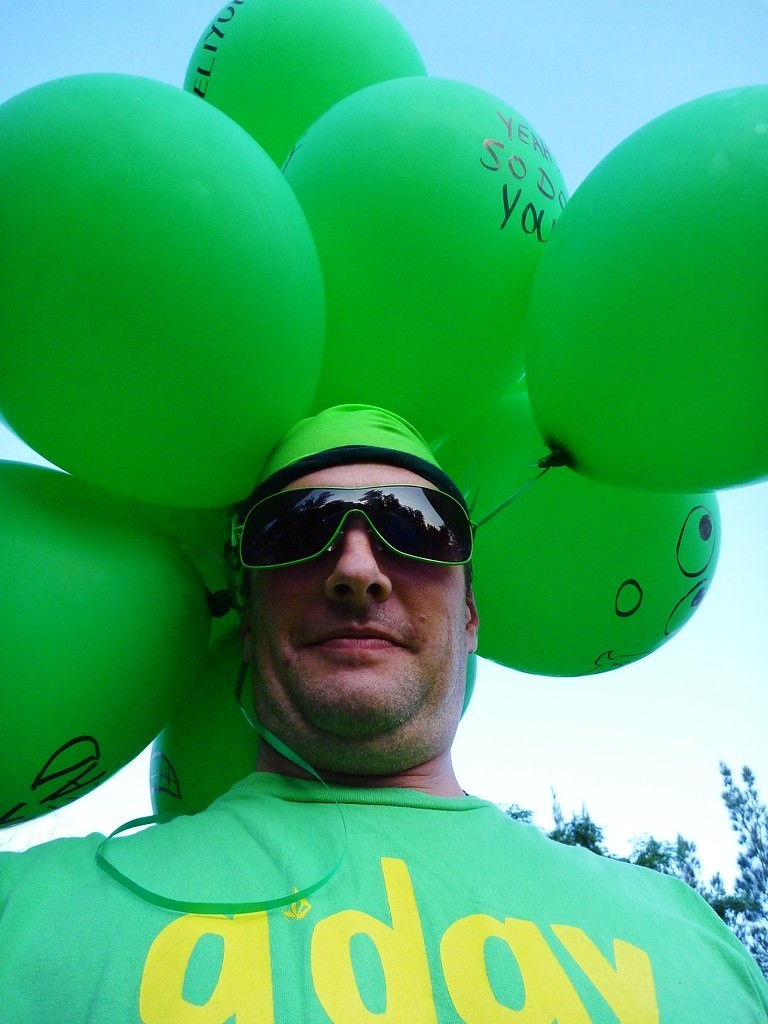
[0,405,768,1024]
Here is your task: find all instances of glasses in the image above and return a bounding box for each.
[231,482,476,571]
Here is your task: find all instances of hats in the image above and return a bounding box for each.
[235,401,470,528]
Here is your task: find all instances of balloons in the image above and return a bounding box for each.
[0,0,768,829]
